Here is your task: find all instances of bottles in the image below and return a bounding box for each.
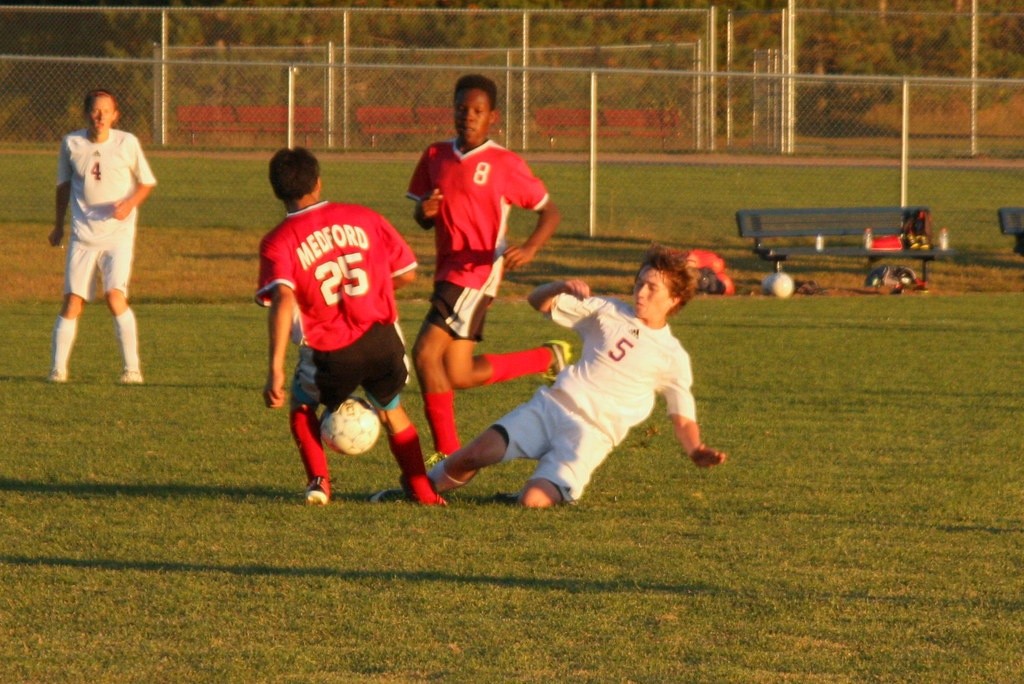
[938,226,949,251]
[863,227,872,252]
[815,233,824,252]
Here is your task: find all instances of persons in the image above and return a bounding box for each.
[366,244,727,509]
[48,88,155,386]
[406,75,572,470]
[254,145,446,510]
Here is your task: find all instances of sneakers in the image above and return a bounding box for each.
[303,478,327,505]
[423,452,449,470]
[541,339,571,384]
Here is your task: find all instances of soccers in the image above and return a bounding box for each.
[765,273,795,298]
[320,394,381,456]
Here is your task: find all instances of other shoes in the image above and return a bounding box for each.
[48,369,68,382]
[371,487,405,502]
[119,371,143,384]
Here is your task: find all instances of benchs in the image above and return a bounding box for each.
[736,205,955,291]
[999,206,1024,257]
